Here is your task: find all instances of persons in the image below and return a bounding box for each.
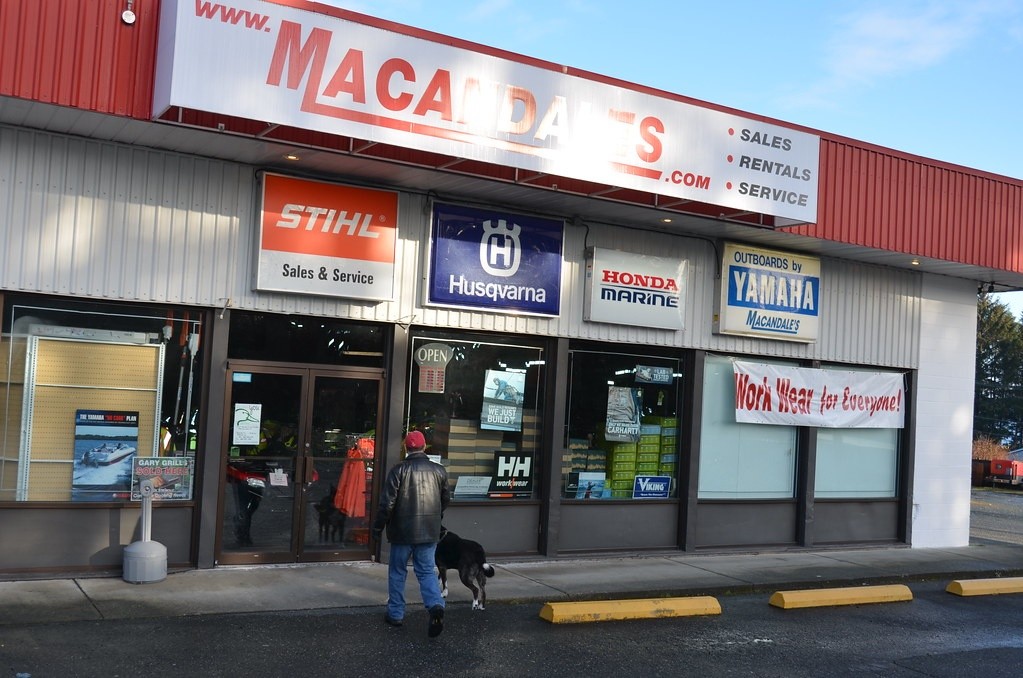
[372,431,451,638]
[227,460,268,549]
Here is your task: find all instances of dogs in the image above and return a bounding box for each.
[434,525,494,610]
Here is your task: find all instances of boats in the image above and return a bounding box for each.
[82,444,136,465]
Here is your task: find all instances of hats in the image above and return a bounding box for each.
[405,431,425,447]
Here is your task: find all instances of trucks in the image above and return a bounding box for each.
[986,459,1023,489]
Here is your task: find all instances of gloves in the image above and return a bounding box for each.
[374,534,380,541]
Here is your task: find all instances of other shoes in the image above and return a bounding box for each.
[384,613,402,626]
[428,606,444,638]
